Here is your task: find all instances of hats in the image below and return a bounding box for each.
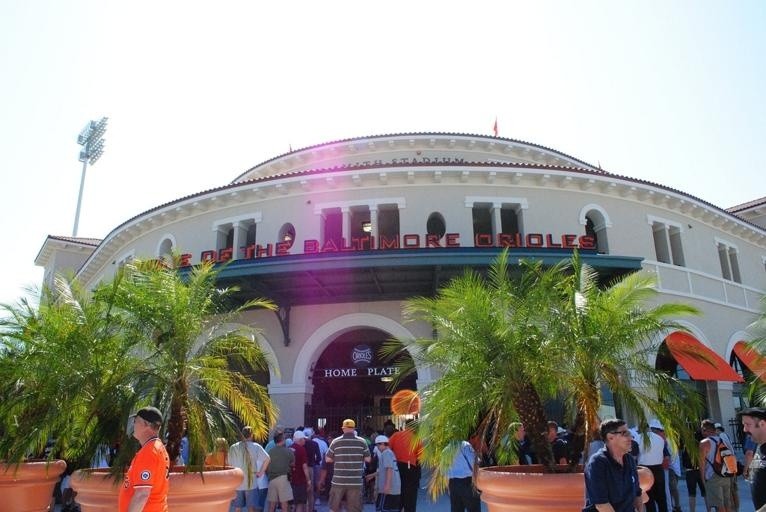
[129,407,163,426]
[649,418,662,429]
[293,419,389,444]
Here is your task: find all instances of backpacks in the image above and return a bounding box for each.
[705,437,737,477]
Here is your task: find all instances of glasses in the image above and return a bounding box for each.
[612,431,630,438]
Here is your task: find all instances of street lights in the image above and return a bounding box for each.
[71,116,108,236]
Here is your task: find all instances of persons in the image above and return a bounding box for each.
[439,425,479,511]
[205,418,424,511]
[117,406,169,511]
[585,404,766,511]
[503,421,526,465]
[89,440,110,467]
[548,421,568,464]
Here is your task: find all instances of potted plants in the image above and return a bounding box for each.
[0,267,79,512]
[379,236,723,512]
[0,244,281,512]
